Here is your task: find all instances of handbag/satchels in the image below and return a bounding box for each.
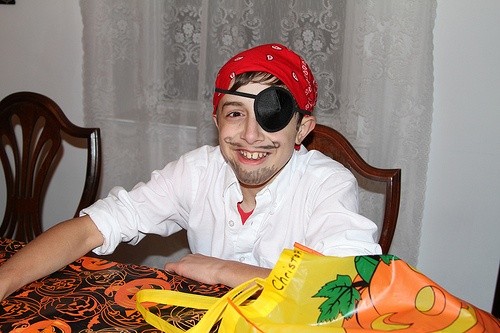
[134,242,500,333]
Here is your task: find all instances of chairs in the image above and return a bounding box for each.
[306,124,402,255]
[0,91,101,243]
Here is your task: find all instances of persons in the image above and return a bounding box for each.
[0,42,383,303]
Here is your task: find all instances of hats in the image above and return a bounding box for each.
[211,44,318,116]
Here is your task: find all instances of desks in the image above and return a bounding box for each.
[0,236,241,333]
